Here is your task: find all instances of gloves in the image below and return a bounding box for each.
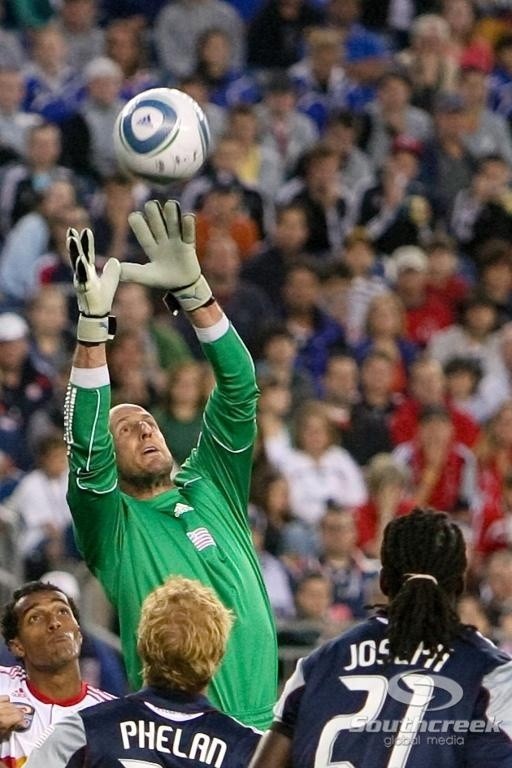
[66,227,120,345]
[122,200,215,314]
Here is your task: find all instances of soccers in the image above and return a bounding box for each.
[114,87,211,184]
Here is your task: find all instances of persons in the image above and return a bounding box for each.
[0,0,512,768]
